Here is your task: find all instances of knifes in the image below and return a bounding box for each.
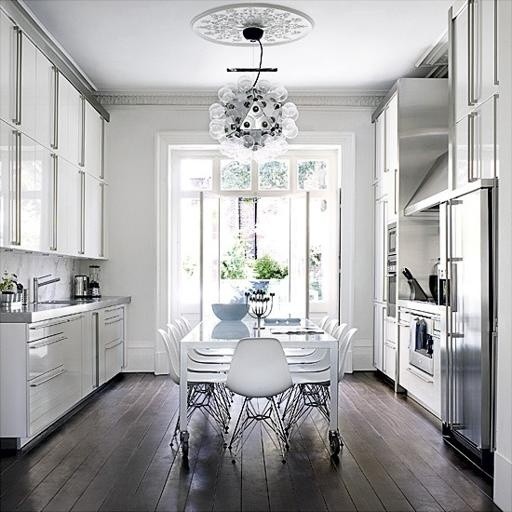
[401,267,412,278]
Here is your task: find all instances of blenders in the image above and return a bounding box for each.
[87,265,103,298]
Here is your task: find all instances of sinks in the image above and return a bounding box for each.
[33,300,74,305]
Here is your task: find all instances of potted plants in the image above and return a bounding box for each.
[250,256,290,291]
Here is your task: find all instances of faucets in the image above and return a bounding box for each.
[33,273,61,305]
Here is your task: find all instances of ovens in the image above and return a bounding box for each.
[405,309,436,377]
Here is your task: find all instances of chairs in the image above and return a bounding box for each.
[157,313,358,465]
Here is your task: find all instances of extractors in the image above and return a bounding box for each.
[403,151,450,221]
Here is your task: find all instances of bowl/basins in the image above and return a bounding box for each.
[209,303,253,322]
[211,321,252,341]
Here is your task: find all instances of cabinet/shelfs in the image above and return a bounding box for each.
[0,305,126,455]
[371,78,448,183]
[448,0,512,189]
[0,0,110,261]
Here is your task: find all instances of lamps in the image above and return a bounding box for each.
[190,3,314,164]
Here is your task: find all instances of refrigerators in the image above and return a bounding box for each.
[437,177,497,457]
